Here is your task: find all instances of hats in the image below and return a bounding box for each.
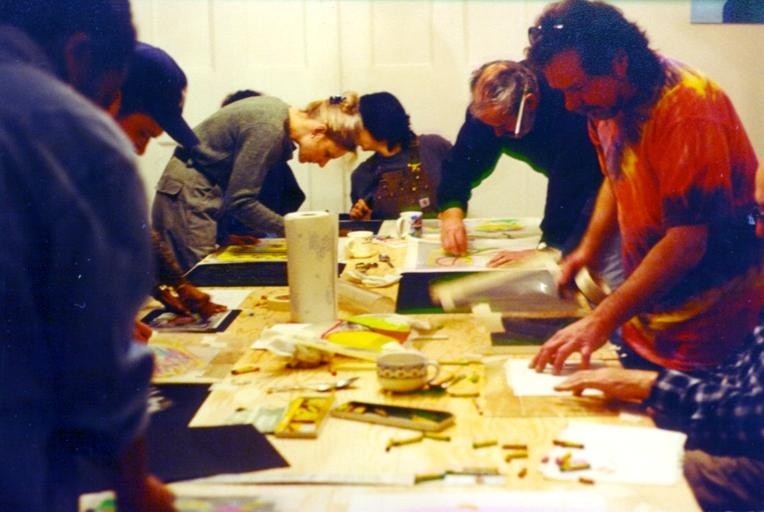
[129,43,199,146]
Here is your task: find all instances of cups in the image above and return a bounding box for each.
[375,353,440,393]
[345,231,373,258]
[396,210,422,242]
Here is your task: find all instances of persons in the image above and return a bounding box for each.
[145,221,228,320]
[149,91,364,274]
[434,57,603,270]
[1,0,176,512]
[528,0,764,376]
[552,160,764,510]
[75,42,200,349]
[221,88,309,246]
[349,89,472,225]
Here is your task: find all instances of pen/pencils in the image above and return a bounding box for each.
[363,193,373,204]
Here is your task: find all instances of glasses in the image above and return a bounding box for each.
[529,23,574,45]
